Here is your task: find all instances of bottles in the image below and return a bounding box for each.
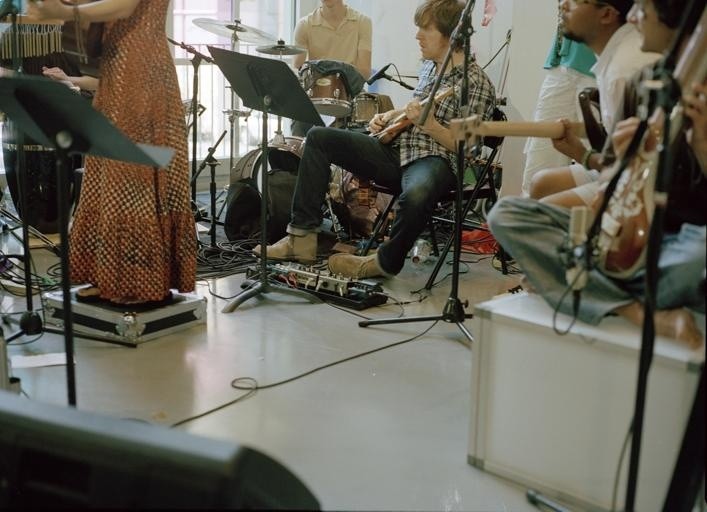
[411,237,432,263]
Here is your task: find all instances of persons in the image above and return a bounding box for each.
[252,0,495,279]
[487,0,707,349]
[290,0,373,135]
[491,34,598,268]
[7,0,197,307]
[0,10,100,233]
[492,0,661,298]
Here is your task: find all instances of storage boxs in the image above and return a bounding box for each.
[465,287,706,512]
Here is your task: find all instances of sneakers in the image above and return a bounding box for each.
[493,285,535,300]
[328,247,394,282]
[252,223,321,264]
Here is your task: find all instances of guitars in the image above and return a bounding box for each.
[589,2,706,279]
[369,86,457,143]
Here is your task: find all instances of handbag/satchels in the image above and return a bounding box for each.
[224,169,298,249]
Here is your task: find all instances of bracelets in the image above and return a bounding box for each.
[582,149,598,172]
[72,6,80,25]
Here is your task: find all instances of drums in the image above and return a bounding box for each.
[299,60,352,117]
[350,95,394,123]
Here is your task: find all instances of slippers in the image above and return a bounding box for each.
[76,285,102,302]
[109,291,172,311]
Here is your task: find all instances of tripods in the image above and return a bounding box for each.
[0,5,144,357]
[356,43,477,343]
[203,44,328,313]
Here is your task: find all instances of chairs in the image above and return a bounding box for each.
[358,106,509,290]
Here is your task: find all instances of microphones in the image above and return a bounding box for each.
[566,202,597,292]
[365,61,393,87]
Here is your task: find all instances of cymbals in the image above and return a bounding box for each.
[193,19,278,44]
[256,42,308,56]
